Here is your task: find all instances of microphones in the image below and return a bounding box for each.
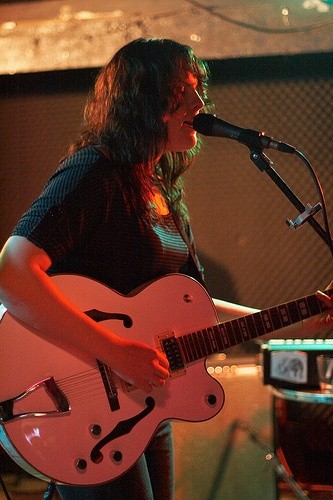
[193,112,297,155]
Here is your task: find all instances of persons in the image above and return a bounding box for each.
[0,36,332,500]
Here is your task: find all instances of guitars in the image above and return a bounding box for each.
[0,268,333,489]
